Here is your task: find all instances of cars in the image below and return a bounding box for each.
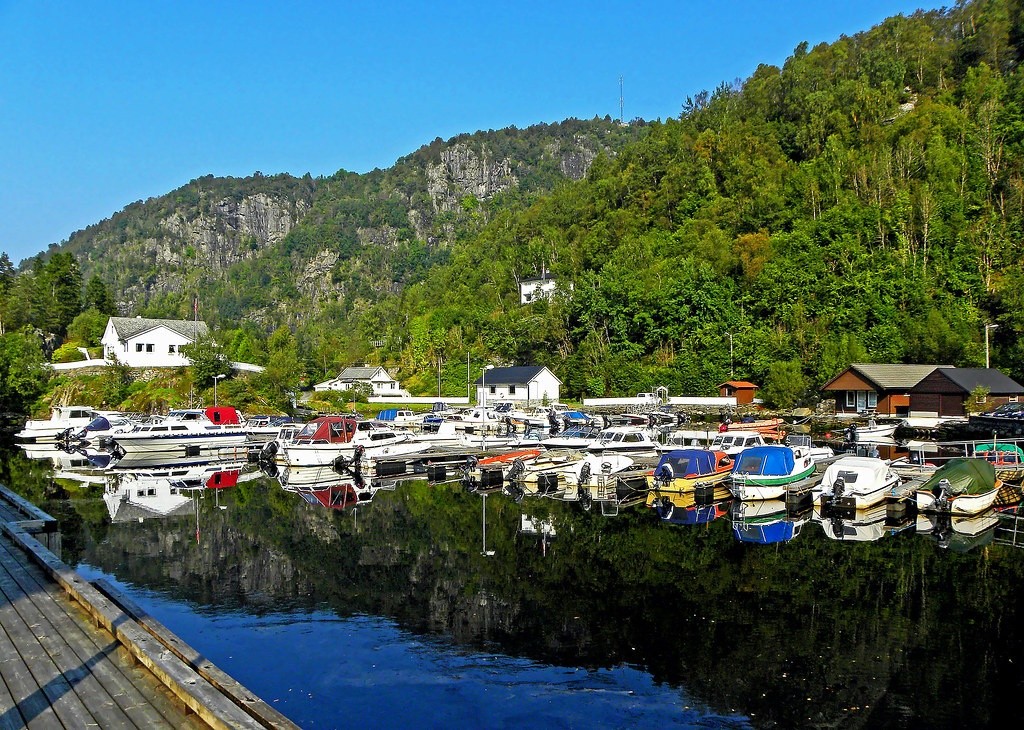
[979,403,1011,417]
[993,402,1024,419]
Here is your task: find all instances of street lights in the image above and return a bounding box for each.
[726,332,733,376]
[986,324,999,371]
[482,364,494,433]
[212,374,227,407]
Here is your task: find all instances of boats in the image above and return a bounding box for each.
[502,480,540,503]
[729,500,813,544]
[646,485,732,524]
[558,483,617,511]
[13,370,1024,517]
[915,504,1004,549]
[606,488,650,510]
[811,501,887,542]
[993,511,1024,551]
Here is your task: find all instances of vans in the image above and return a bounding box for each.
[637,393,663,406]
